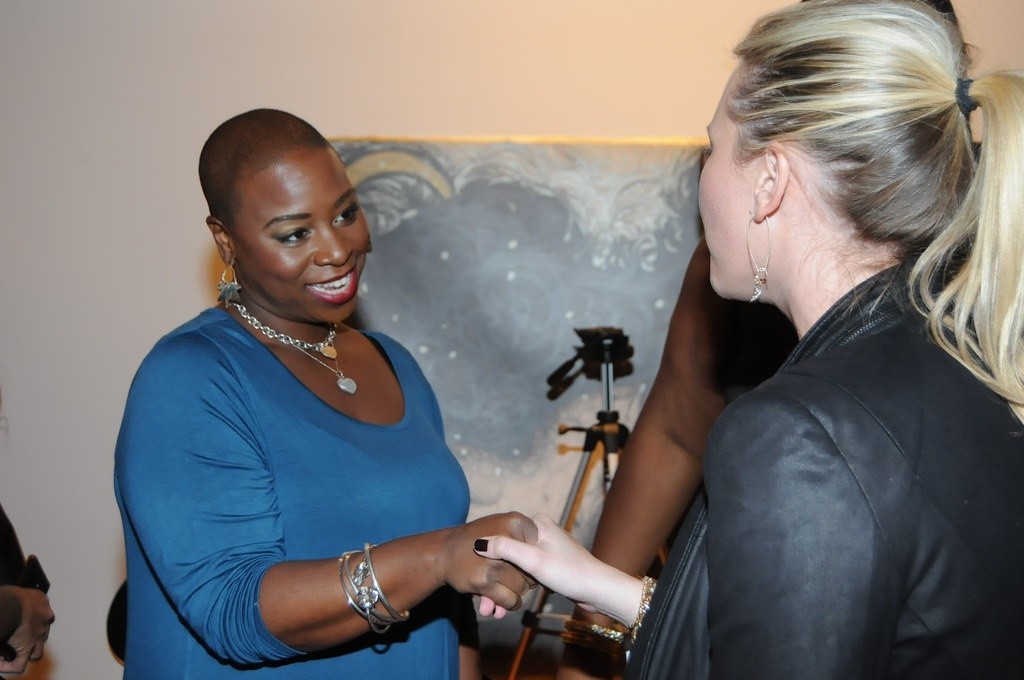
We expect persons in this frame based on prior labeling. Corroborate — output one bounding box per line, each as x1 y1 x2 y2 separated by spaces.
474 0 1024 680
112 108 537 680
0 506 55 680
556 0 959 680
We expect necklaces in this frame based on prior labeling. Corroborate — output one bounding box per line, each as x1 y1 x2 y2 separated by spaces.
231 301 357 396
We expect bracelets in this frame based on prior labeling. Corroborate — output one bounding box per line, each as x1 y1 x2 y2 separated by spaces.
335 542 409 635
561 574 659 658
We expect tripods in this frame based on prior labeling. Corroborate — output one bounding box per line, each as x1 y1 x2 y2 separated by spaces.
508 325 673 680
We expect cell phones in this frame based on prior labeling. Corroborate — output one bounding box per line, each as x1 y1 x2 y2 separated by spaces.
0 555 50 662
